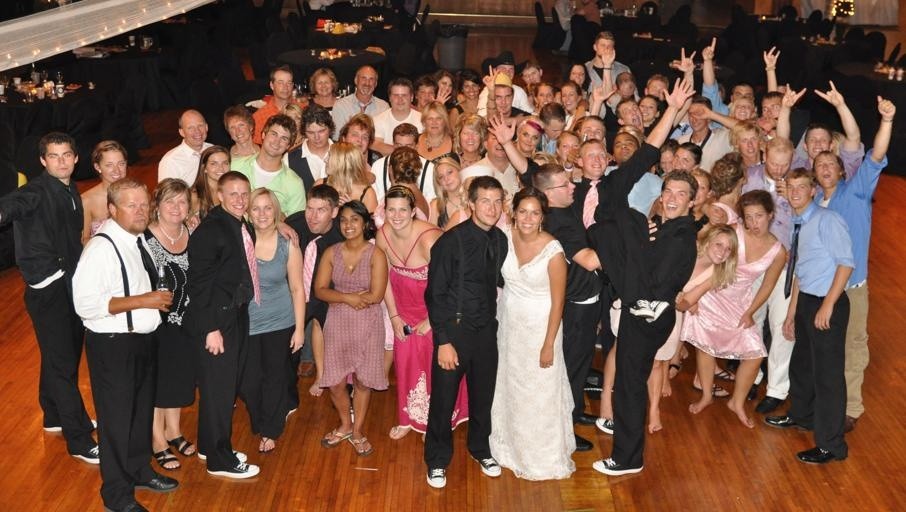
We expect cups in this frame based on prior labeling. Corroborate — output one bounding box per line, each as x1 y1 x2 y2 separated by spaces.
324 23 331 33
0 65 83 103
776 177 785 195
887 67 904 82
142 37 152 48
128 36 135 47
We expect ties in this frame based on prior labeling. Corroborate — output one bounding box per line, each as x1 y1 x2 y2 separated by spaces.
580 177 603 232
300 234 324 305
781 223 803 301
134 236 170 328
239 221 262 307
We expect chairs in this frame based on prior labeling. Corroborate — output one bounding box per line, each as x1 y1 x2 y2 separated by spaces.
533 2 906 179
187 0 439 107
1 20 187 196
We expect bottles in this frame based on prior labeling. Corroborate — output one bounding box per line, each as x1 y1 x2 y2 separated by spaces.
574 133 589 169
155 264 171 309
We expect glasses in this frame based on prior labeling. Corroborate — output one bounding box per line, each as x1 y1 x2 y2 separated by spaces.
542 180 569 191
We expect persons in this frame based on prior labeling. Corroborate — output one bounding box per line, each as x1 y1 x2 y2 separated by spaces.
1 131 102 467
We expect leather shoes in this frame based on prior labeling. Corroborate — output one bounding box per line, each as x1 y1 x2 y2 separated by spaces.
793 446 848 467
744 381 759 403
133 470 181 494
754 394 787 415
571 431 594 452
101 499 149 512
762 413 812 432
571 411 601 426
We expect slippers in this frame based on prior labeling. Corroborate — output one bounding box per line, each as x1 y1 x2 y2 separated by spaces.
321 426 355 449
348 432 375 456
711 366 737 381
388 423 413 441
257 435 276 455
689 383 730 398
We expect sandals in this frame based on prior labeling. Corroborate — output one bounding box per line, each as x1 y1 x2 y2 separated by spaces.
150 447 182 471
166 436 196 458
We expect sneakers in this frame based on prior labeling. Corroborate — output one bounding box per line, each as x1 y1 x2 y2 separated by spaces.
69 443 102 465
841 409 857 435
425 465 447 489
469 453 503 479
42 418 99 433
196 446 248 464
204 462 261 480
595 415 617 434
591 456 644 478
